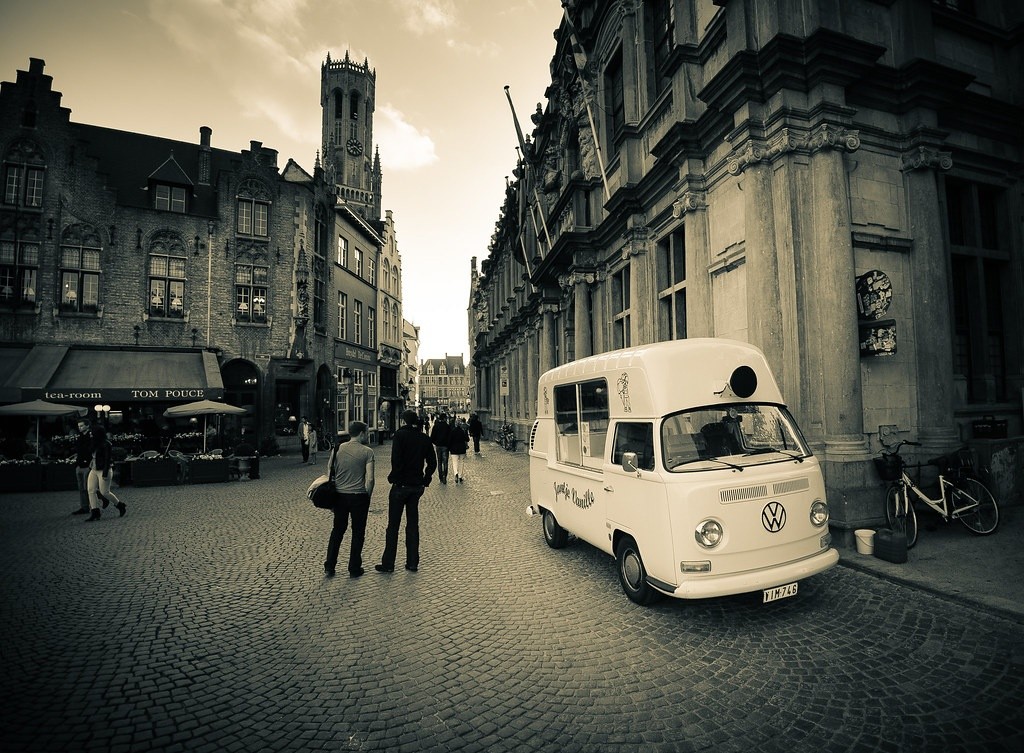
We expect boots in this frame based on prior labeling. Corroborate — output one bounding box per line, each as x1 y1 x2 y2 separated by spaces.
84 508 101 522
116 501 127 517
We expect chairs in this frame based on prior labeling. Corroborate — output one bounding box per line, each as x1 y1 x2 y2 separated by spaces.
700 423 740 457
619 425 654 470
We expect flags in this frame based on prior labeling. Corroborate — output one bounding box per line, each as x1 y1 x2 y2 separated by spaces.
564 5 592 83
505 90 538 266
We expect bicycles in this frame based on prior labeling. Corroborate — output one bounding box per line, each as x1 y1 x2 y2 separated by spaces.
872 433 999 548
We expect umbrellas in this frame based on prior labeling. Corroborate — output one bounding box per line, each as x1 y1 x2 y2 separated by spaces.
163 400 247 454
0 399 88 458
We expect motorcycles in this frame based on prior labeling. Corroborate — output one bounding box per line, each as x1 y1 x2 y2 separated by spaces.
500 422 516 451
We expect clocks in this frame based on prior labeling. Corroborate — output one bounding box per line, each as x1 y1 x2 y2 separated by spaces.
346 138 363 156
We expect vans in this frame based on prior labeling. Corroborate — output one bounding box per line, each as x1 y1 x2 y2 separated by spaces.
528 336 844 609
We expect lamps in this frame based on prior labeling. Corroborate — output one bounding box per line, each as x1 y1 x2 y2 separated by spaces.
409 378 413 384
343 368 351 389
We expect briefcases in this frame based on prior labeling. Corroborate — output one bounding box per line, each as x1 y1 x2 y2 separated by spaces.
972 415 1008 438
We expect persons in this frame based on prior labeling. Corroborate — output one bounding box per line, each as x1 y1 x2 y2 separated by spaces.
71 419 127 522
378 416 385 445
203 423 217 437
297 416 318 465
375 410 437 572
422 407 484 484
324 420 375 578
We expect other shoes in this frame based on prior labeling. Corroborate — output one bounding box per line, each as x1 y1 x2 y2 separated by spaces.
350 568 364 577
375 565 394 572
455 473 459 483
308 461 316 465
440 479 447 485
405 564 417 571
325 568 336 574
474 451 481 456
459 478 463 483
71 508 90 515
102 499 109 509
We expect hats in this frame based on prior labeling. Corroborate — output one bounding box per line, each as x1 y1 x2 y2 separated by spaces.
400 410 418 425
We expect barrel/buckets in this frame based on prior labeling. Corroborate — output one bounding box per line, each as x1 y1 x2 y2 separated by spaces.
854 529 876 555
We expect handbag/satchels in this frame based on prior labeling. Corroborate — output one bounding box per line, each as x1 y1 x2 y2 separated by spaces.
307 475 336 510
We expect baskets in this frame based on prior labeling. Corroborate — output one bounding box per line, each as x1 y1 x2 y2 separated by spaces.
873 454 902 481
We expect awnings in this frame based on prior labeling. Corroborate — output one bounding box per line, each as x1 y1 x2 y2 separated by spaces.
0 345 224 400
